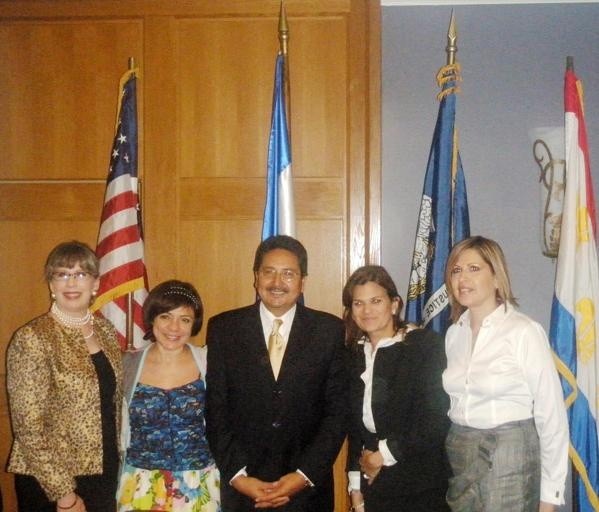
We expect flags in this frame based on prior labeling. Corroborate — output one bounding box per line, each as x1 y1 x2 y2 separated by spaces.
90 68 153 349
547 68 599 512
260 53 297 242
404 61 470 335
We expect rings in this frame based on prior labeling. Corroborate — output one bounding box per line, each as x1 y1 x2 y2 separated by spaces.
363 473 369 480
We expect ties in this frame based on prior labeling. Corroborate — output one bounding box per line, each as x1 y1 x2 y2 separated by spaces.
266 318 289 383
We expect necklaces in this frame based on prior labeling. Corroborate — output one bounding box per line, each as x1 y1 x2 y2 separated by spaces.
50 301 96 340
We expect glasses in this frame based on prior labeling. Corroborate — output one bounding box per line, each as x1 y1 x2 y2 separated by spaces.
50 270 97 284
254 267 307 283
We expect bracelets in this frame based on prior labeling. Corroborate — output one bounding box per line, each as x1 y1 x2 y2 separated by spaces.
304 478 312 488
59 494 78 510
347 503 365 512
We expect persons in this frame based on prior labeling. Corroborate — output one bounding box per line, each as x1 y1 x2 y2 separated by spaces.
7 240 122 512
118 279 222 512
343 266 454 511
442 235 568 512
207 234 345 512
